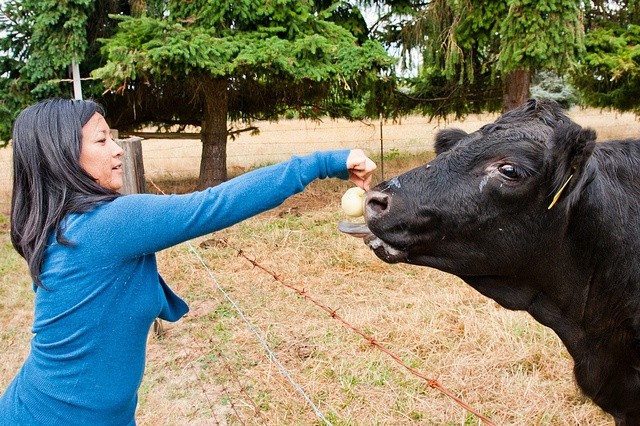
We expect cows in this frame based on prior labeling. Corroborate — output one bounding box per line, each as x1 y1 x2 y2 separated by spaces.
337 98 639 426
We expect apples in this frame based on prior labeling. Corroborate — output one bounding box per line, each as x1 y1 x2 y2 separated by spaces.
341 186 366 217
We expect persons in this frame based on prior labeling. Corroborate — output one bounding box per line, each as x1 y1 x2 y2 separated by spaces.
0 97 377 426
